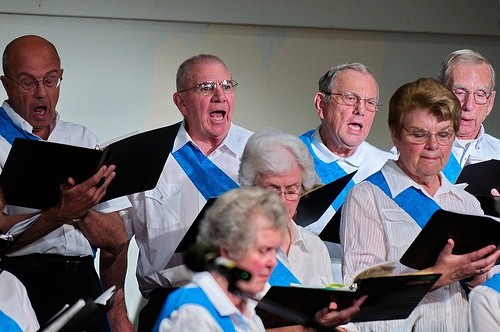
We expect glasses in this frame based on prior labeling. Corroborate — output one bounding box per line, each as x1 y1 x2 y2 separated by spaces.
259 186 302 201
323 92 383 112
402 124 455 146
7 74 62 91
451 88 493 104
179 80 239 96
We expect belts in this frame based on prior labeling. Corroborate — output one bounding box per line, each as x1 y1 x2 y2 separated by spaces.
12 260 93 274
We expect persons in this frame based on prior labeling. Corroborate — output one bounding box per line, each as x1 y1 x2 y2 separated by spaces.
292 49 500 332
0 35 360 332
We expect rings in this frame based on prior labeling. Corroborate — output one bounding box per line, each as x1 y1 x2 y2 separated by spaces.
480 268 484 274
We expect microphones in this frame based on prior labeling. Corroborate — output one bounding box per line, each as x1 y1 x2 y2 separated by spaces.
184 247 252 281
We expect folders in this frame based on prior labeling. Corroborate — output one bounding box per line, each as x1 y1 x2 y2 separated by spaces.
255 269 442 332
37 255 118 332
0 214 42 258
399 209 500 281
175 170 358 253
318 183 471 244
0 121 183 211
455 159 500 218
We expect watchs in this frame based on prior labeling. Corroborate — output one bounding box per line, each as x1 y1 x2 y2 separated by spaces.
70 210 89 226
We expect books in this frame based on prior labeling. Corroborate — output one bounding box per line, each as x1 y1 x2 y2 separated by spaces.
399 209 500 271
36 285 119 332
254 258 443 329
318 204 344 244
161 170 358 270
399 208 500 270
0 120 183 211
455 154 500 195
0 214 41 258
